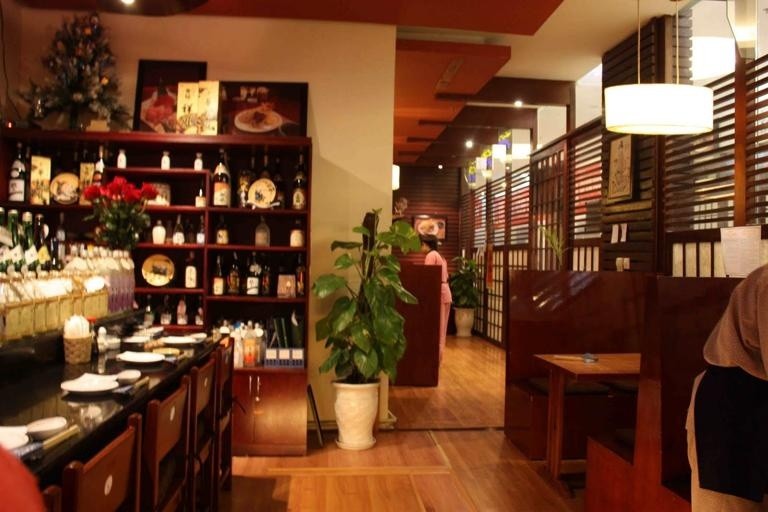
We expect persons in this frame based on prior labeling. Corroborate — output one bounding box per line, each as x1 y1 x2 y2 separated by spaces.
694 262 768 503
419 234 452 363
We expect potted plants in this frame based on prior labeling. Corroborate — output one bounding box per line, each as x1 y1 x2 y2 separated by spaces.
312 210 418 453
449 256 480 338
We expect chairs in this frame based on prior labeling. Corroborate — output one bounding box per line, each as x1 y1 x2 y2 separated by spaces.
217 336 235 497
43 485 62 512
191 350 218 512
144 374 193 512
61 413 144 512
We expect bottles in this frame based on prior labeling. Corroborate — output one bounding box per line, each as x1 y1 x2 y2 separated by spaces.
194 295 204 326
227 250 241 295
160 150 171 171
259 153 271 180
143 294 156 325
159 295 173 325
245 251 262 296
193 152 204 171
212 147 232 208
254 215 271 247
288 219 306 248
172 214 186 245
116 148 127 169
196 215 205 244
211 317 267 369
152 220 167 244
183 250 198 289
194 175 206 208
86 316 99 354
92 143 106 204
0 207 6 273
55 211 67 262
8 142 27 203
295 251 306 296
216 215 231 244
290 151 308 210
21 211 40 272
64 245 136 319
51 238 64 271
0 271 64 347
175 296 189 326
273 156 286 208
7 209 24 272
33 213 52 271
211 255 225 295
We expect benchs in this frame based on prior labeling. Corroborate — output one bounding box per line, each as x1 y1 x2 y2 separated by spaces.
504 267 654 461
648 274 746 511
583 275 648 511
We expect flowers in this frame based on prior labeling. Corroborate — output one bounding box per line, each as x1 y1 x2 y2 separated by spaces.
83 175 158 251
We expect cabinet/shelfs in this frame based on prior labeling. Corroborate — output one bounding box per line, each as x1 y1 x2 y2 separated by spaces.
1 124 311 457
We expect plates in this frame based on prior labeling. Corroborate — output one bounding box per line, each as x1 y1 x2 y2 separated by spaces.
162 336 195 344
1 426 29 450
234 108 283 133
50 173 80 204
248 177 277 204
119 352 164 364
141 255 175 287
61 379 119 394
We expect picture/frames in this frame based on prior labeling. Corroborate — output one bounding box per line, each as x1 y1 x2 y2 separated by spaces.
604 135 635 204
412 214 448 242
218 80 309 137
133 58 208 134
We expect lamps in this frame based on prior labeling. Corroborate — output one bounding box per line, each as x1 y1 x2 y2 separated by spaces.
604 1 715 137
